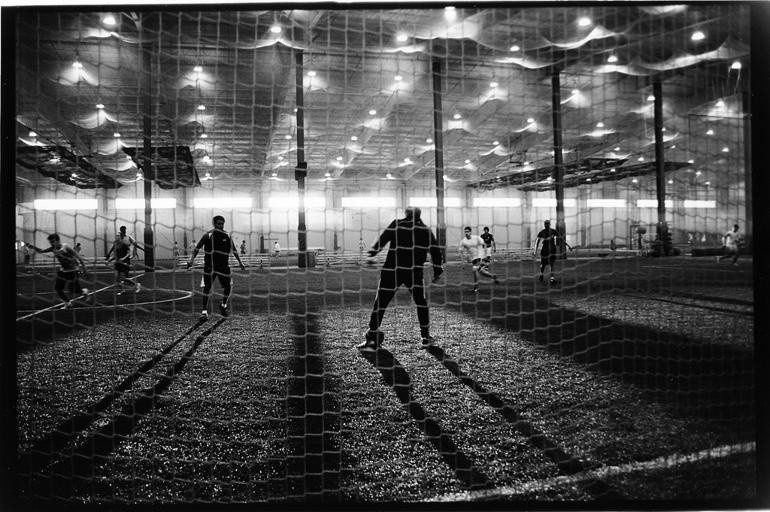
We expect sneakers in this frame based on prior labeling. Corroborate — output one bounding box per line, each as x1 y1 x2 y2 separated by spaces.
356 328 384 352
473 265 556 293
202 300 228 321
422 336 438 348
60 282 143 310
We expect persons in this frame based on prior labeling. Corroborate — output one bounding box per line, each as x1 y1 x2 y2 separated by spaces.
273 241 281 257
103 225 152 285
25 233 89 312
130 242 140 261
23 245 32 274
73 243 85 267
459 225 499 295
172 241 181 257
184 215 245 322
531 219 574 285
480 227 495 271
188 239 196 255
353 204 443 353
717 223 745 266
358 237 365 255
113 231 143 296
240 240 247 254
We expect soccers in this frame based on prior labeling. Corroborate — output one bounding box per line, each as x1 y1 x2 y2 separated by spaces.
366 328 384 345
